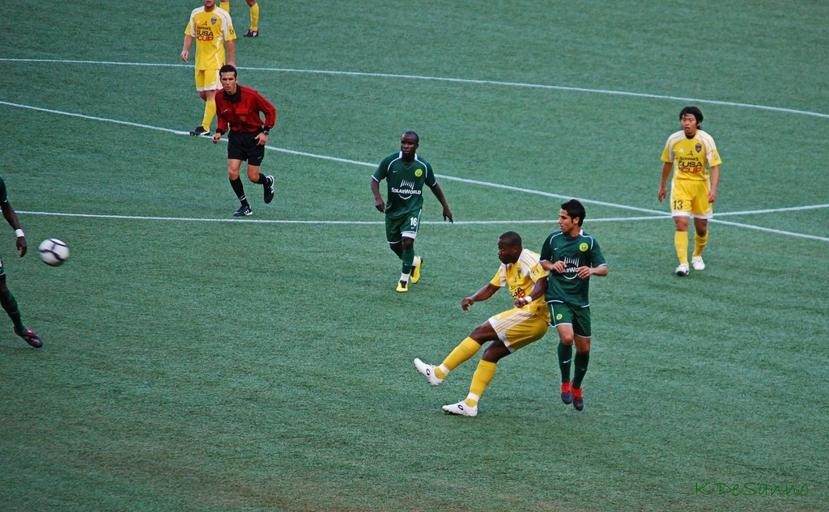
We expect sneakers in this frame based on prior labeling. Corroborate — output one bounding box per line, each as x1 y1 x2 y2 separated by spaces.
442 400 477 416
263 175 275 203
190 126 210 136
244 29 258 37
414 358 444 386
396 256 423 292
14 326 42 347
233 205 252 216
692 256 705 270
561 382 583 410
675 264 689 276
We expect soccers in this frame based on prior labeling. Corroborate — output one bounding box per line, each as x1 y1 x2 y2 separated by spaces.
37 238 70 267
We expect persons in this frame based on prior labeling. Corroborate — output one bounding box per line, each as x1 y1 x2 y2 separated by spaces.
212 65 276 216
0 178 44 349
413 231 550 417
181 1 237 136
370 130 453 292
219 0 259 37
658 106 722 276
539 199 609 411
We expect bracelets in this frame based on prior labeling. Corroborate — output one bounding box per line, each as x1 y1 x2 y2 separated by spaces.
14 228 23 237
524 295 532 303
